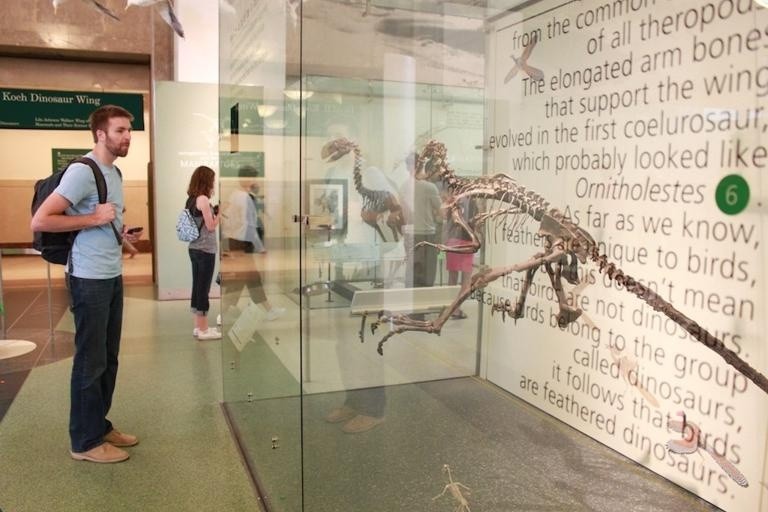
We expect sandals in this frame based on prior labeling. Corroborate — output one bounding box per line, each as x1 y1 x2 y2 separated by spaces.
450 310 467 318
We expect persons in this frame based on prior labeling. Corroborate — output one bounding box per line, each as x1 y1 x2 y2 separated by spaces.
216 165 286 326
322 120 400 435
245 182 264 253
399 152 443 321
445 199 479 320
184 166 228 340
30 105 138 464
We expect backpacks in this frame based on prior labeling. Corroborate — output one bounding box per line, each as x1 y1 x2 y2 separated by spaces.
176 197 205 241
32 157 122 265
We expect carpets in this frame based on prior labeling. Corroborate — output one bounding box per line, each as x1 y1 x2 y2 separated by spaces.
0 280 303 511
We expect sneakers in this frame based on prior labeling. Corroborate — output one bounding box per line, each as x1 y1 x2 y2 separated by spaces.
193 328 217 336
267 307 286 320
198 329 222 340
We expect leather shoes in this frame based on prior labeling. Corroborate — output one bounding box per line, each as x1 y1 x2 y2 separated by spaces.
105 428 138 446
342 415 385 433
326 407 356 422
71 442 129 463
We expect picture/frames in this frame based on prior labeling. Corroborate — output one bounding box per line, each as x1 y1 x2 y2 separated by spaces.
304 179 347 231
